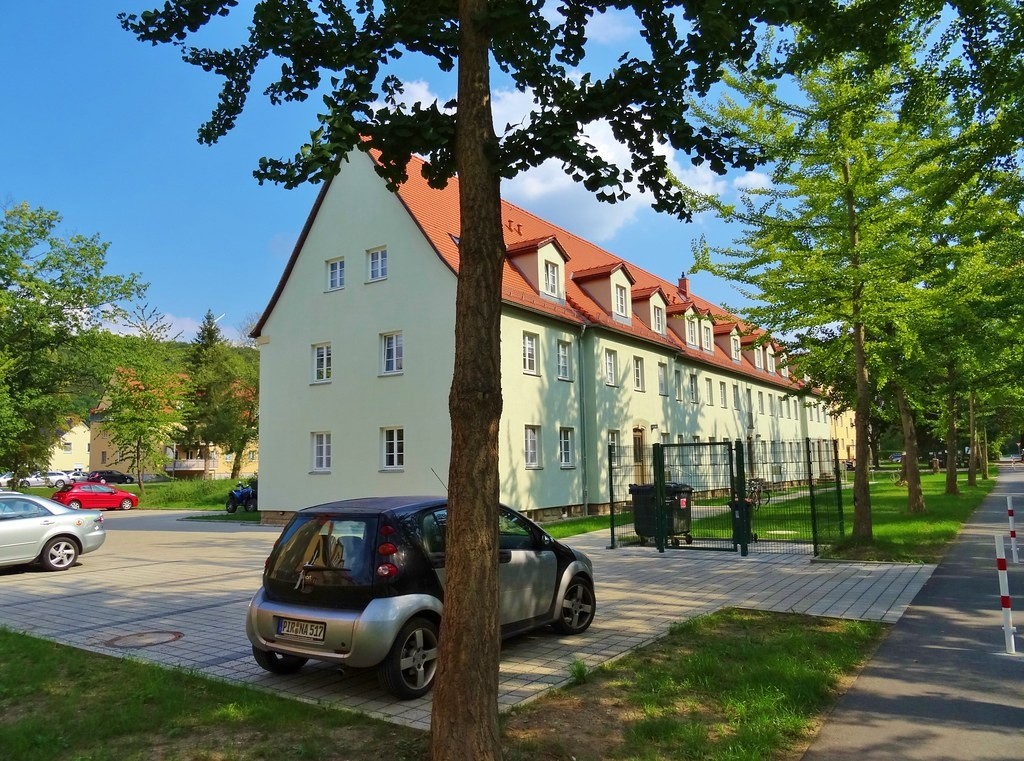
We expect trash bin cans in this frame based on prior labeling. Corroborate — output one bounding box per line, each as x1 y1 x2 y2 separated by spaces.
628 483 694 548
727 499 759 545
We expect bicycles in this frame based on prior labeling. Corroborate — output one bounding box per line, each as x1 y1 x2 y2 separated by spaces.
744 478 770 511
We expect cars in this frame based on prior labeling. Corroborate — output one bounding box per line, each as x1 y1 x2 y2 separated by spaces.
50 482 139 512
18 470 70 489
87 469 134 486
62 471 90 484
1 491 107 571
243 494 597 700
0 472 23 488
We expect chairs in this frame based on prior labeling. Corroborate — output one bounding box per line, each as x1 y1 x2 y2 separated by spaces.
319 534 336 565
337 536 361 559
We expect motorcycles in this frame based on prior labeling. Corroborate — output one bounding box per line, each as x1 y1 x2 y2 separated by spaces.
226 483 258 514
